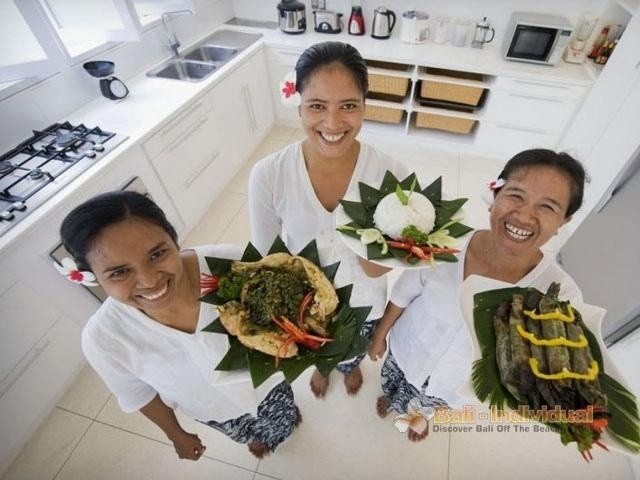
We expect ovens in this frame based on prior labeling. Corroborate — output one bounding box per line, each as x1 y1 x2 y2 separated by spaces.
48 175 174 307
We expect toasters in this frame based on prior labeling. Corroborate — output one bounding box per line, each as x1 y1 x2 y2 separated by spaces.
312 10 343 34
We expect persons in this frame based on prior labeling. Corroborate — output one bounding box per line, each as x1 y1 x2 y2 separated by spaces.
248 42 410 399
61 191 302 460
369 149 585 441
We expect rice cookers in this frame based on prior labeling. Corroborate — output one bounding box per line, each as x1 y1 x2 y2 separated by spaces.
276 0 307 34
401 11 432 44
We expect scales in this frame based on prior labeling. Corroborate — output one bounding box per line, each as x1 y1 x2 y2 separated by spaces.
84 61 129 101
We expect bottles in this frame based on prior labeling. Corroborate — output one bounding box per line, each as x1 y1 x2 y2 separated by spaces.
587 21 627 65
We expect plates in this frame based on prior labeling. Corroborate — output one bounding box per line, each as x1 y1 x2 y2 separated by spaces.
457 274 638 415
335 180 473 270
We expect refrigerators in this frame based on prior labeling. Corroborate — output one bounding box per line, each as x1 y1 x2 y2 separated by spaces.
556 144 640 350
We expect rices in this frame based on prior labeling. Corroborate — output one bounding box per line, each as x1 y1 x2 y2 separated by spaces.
373 189 436 242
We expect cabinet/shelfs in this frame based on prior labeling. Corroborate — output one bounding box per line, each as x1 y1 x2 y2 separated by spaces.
0 50 275 479
266 46 498 145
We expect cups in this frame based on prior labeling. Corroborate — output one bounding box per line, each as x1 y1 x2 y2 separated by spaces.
436 14 472 48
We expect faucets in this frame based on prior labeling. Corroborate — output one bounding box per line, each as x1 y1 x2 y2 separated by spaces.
161 8 197 42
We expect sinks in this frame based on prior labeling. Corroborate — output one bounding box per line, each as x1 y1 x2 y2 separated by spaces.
156 61 217 82
185 45 238 61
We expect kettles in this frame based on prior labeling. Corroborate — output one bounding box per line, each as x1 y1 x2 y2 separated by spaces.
371 6 396 39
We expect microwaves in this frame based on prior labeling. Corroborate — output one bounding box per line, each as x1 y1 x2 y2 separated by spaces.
501 12 573 67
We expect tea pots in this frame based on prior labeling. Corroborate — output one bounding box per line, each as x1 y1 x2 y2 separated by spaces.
472 16 494 48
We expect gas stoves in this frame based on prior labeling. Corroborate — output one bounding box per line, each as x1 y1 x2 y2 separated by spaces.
0 121 116 232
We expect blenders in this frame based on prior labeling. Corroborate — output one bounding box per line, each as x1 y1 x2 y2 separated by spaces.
562 14 599 65
350 0 365 36
